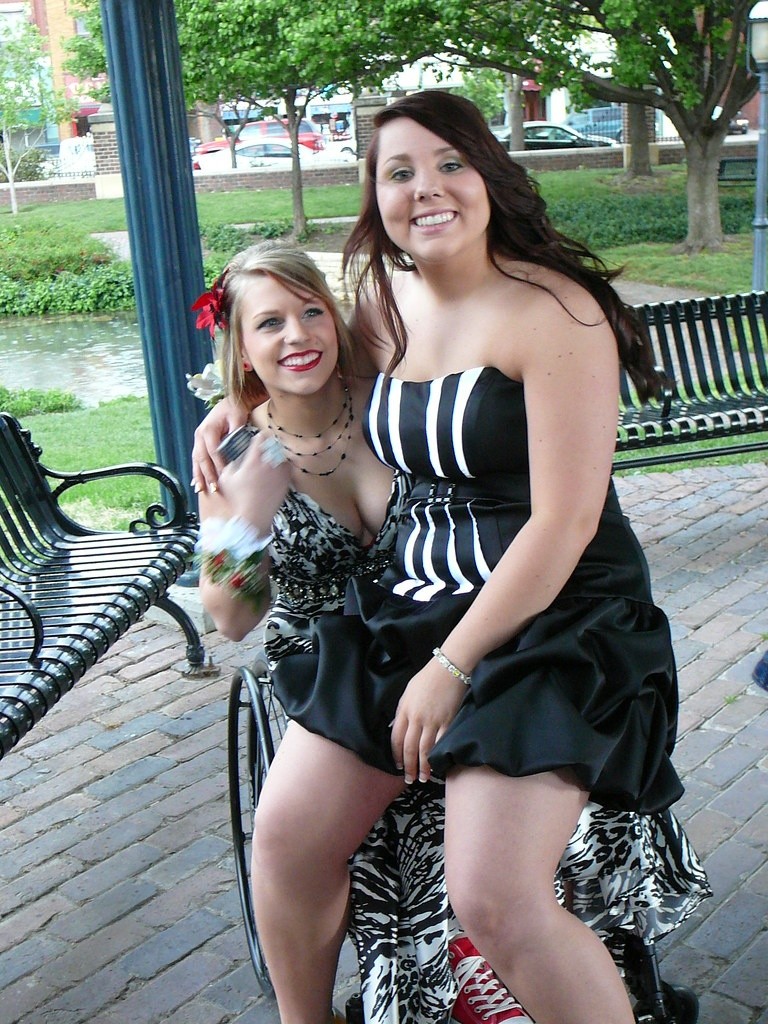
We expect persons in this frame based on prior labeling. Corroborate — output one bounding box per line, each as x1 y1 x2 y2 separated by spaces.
190 90 688 1023
192 238 717 1023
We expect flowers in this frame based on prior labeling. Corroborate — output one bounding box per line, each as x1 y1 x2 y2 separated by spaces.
191 280 227 339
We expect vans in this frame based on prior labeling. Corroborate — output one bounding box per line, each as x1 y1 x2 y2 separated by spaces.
34 144 60 159
555 106 664 143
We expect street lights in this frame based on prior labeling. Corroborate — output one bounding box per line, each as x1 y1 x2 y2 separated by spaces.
746 1 768 292
36 64 49 145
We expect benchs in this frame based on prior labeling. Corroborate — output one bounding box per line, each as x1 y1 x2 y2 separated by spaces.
611 290 768 475
0 411 220 764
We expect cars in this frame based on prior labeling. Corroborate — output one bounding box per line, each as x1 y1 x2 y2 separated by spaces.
334 139 357 162
491 121 618 152
713 109 749 134
191 119 326 156
191 138 324 171
327 120 352 142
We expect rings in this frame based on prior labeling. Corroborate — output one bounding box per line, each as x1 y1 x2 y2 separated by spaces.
258 437 285 468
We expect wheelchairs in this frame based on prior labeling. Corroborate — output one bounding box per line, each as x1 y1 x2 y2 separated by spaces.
228 651 700 1024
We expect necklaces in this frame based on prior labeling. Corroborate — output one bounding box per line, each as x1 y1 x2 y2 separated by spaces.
267 377 354 477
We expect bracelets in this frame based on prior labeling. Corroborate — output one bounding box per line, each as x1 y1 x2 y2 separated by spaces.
431 646 472 686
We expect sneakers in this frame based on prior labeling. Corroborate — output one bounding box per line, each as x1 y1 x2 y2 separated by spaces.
448 932 537 1024
